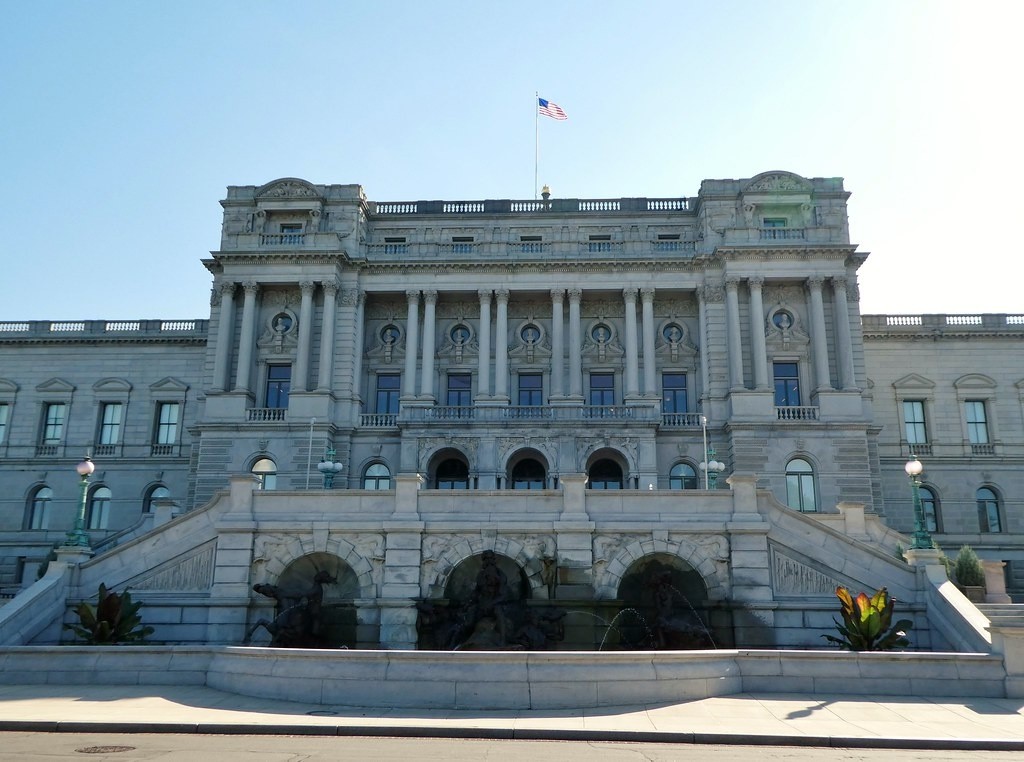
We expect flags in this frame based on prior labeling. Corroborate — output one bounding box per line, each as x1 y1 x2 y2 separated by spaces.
539 97 568 120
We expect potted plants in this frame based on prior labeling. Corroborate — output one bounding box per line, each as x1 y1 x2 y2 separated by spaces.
955 544 986 603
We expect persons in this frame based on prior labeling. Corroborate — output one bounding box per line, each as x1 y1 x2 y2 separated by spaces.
448 549 515 650
276 569 338 639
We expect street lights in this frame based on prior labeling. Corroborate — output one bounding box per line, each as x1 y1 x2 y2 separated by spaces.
905 453 936 551
59 454 97 548
317 459 345 489
305 415 318 487
699 415 711 490
699 459 725 492
